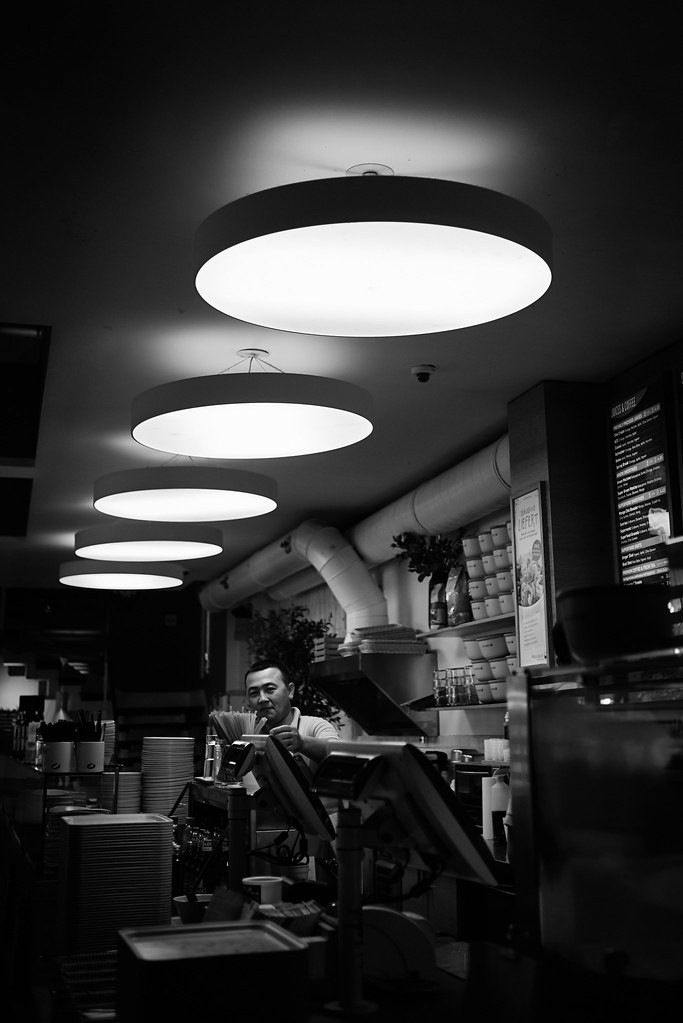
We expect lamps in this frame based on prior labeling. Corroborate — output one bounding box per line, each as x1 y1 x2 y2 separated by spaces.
59 557 187 592
91 465 279 527
70 519 226 564
175 170 562 341
128 366 378 466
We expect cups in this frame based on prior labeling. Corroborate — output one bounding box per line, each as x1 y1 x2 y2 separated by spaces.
75 741 106 772
44 741 75 773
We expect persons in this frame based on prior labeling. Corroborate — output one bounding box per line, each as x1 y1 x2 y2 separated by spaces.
243 658 341 857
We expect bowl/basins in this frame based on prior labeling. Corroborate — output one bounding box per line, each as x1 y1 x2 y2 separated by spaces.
433 518 519 707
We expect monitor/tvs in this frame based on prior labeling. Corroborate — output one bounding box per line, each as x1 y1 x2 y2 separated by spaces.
397 744 503 886
261 736 336 841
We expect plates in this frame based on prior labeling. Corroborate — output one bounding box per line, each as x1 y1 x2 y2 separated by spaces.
101 737 195 827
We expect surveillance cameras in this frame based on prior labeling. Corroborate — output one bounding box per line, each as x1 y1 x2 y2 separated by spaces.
182 569 191 576
411 365 436 383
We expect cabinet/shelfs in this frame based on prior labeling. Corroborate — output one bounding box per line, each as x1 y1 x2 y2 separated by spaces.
406 607 517 717
